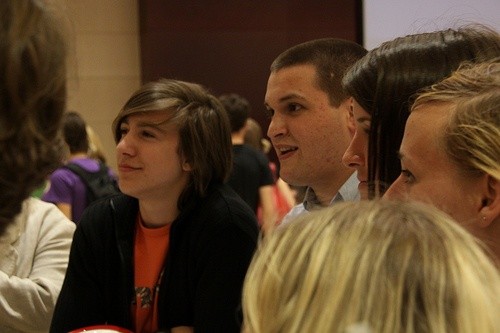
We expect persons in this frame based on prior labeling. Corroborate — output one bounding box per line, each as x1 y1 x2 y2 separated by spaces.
48 81 262 333
0 0 500 333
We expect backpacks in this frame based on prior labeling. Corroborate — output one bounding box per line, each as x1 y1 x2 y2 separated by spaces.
62 158 122 211
